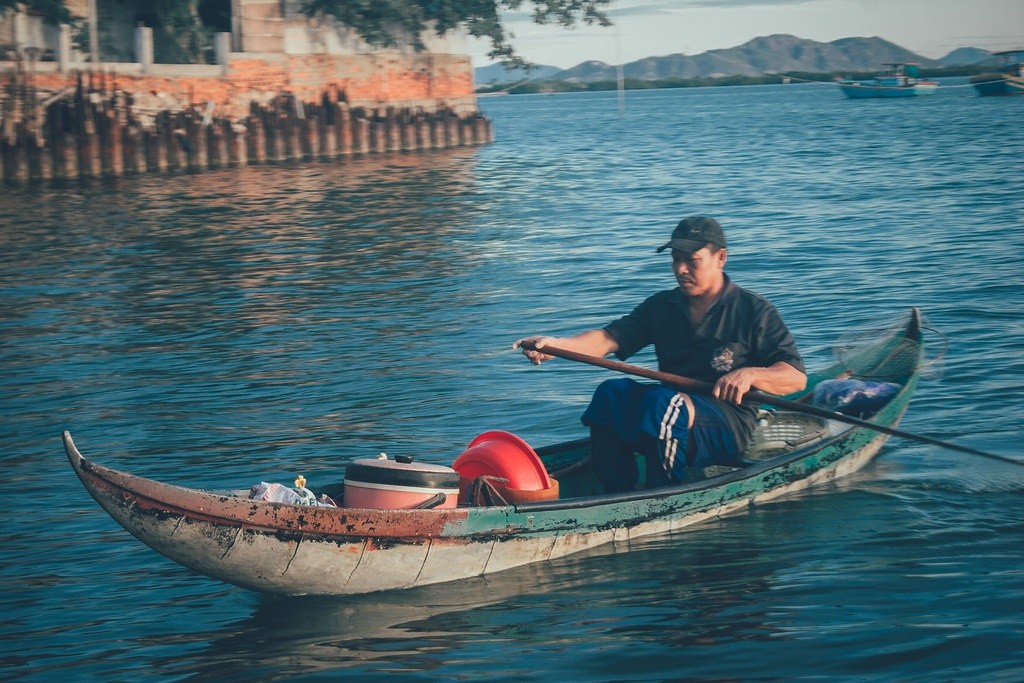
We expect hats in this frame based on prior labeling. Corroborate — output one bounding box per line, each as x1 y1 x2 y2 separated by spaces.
656 215 727 254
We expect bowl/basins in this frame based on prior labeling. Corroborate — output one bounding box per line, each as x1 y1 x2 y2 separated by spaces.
451 430 551 490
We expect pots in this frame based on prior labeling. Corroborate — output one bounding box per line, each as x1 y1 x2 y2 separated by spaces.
344 455 460 509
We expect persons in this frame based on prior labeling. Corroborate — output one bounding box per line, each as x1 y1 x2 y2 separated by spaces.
513 215 809 493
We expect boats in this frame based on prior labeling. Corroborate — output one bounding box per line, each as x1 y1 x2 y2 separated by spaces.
63 306 926 598
837 62 940 97
970 49 1024 96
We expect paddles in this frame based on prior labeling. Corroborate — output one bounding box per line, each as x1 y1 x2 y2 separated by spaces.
522 340 1023 468
